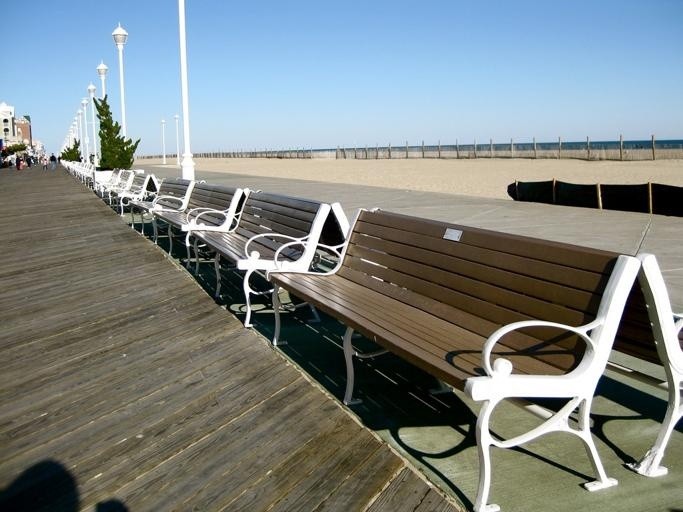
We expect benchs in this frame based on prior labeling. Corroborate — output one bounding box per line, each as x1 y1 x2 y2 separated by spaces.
55 157 240 268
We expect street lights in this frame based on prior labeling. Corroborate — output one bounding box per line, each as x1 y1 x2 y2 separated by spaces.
159 120 167 165
97 59 108 104
112 21 128 151
87 82 97 167
61 98 89 166
174 115 182 169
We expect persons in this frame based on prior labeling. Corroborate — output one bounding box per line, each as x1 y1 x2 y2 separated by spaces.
7 153 60 171
